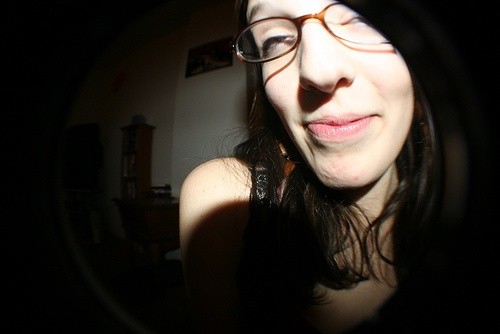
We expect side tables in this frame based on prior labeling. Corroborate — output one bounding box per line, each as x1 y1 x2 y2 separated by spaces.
114 196 181 259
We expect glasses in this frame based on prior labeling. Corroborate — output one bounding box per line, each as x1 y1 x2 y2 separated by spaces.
232 3 392 64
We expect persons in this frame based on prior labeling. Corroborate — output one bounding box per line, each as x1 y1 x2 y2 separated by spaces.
180 0 485 333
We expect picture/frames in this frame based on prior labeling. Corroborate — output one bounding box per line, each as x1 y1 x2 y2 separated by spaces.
185 34 233 78
